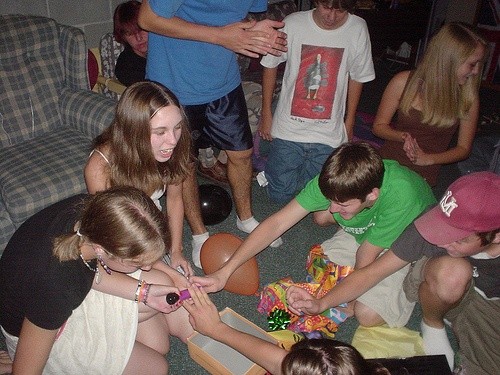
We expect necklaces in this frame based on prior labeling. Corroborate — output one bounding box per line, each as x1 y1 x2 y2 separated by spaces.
79 253 102 286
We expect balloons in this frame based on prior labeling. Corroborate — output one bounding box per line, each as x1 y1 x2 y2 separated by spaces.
200 233 260 299
196 184 233 225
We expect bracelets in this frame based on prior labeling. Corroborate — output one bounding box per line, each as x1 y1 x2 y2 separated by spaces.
134 280 152 305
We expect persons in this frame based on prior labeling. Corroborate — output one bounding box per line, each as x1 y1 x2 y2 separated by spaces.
186 139 444 331
285 170 499 374
81 78 199 356
180 279 397 374
0 184 178 375
257 1 377 207
368 20 490 200
137 1 288 271
112 0 262 185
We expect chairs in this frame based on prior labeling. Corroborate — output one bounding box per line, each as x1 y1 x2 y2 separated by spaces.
96 33 127 101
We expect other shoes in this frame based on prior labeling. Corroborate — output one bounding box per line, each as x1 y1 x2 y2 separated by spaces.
197 160 231 186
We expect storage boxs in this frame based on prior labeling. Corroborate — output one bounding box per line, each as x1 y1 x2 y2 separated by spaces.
187 307 280 375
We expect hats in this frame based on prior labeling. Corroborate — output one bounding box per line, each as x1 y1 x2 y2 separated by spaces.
414 171 500 245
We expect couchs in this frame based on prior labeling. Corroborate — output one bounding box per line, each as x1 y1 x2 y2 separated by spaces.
0 14 119 259
